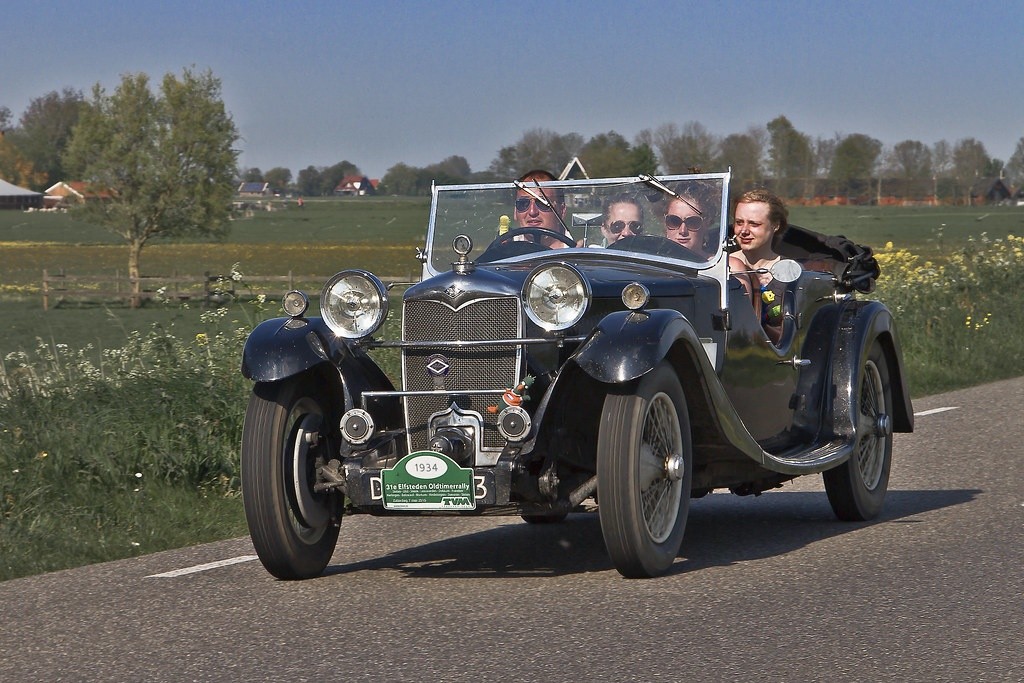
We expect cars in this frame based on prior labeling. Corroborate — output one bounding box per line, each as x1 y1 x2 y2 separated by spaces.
239 167 916 580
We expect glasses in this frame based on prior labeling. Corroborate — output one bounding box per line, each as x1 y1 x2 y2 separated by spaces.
664 214 707 231
605 220 643 234
515 196 558 212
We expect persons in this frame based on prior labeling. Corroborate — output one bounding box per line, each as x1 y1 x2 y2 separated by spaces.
584 193 647 251
487 169 583 250
656 168 754 306
729 189 805 343
